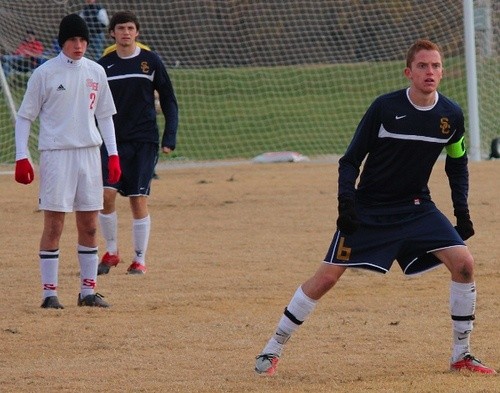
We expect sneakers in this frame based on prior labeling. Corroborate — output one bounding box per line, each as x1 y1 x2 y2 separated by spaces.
253 338 286 376
77 292 111 309
126 261 148 274
97 248 120 275
448 353 497 375
39 295 65 309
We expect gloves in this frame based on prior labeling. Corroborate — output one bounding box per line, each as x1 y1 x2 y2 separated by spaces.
15 159 34 184
452 210 476 240
336 198 358 237
106 154 121 184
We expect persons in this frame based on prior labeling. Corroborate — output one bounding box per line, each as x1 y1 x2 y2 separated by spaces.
79 0 109 61
255 41 495 376
15 14 121 309
95 9 179 274
10 30 44 72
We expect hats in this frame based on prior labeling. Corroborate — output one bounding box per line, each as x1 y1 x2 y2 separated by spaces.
58 13 89 49
